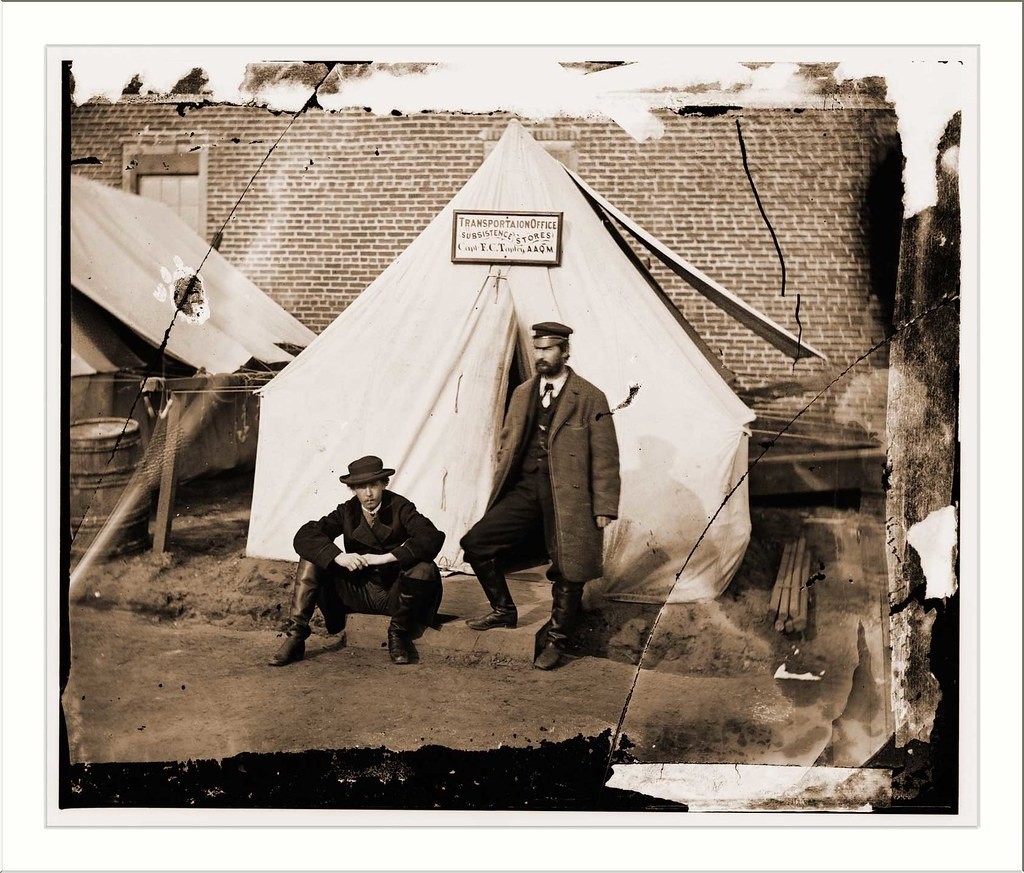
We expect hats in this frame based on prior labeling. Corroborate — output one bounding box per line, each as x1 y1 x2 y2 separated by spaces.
531 322 574 348
339 455 396 485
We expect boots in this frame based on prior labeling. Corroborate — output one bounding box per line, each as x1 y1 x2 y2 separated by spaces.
387 573 433 664
465 558 518 630
268 557 325 665
535 584 586 670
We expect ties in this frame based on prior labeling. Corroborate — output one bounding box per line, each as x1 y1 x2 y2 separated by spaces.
542 383 554 409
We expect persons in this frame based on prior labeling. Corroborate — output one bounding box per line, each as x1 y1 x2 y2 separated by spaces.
462 321 622 670
265 456 445 663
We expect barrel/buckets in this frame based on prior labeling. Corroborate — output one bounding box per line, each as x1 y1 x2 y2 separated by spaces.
70 416 152 567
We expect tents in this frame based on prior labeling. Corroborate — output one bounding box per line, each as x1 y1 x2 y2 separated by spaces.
243 120 827 603
63 172 320 484
